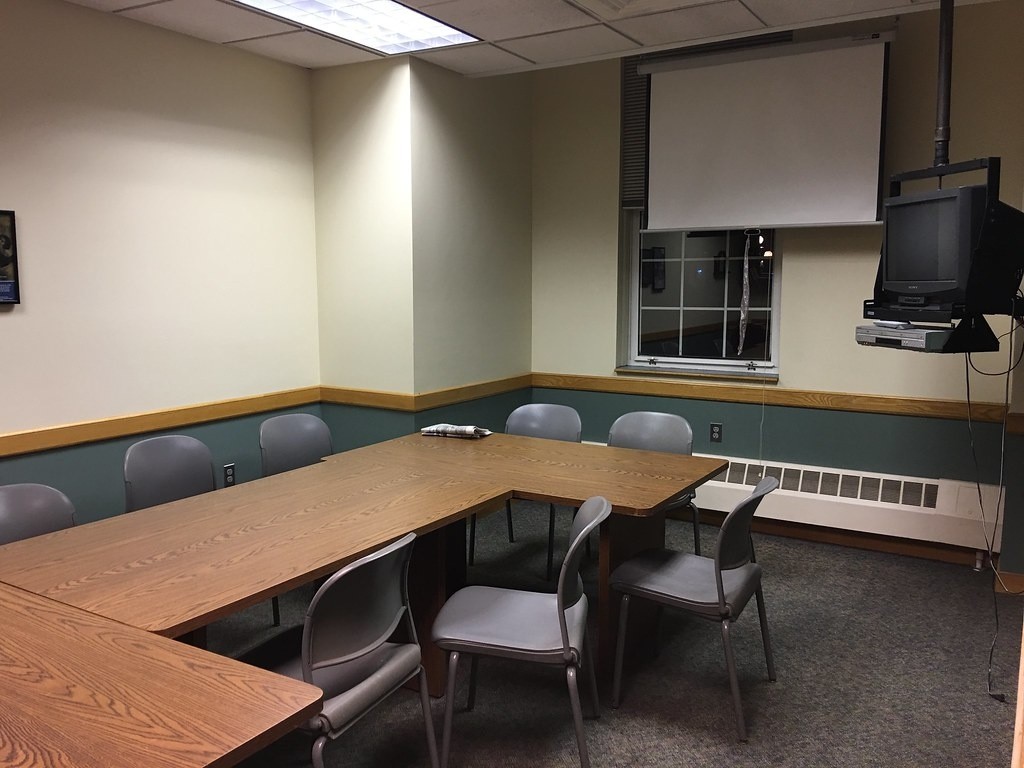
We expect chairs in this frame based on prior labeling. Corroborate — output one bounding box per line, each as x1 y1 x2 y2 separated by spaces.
259 413 332 600
0 483 75 544
228 533 440 768
429 496 612 768
607 477 782 743
469 403 583 582
124 434 281 624
572 411 701 562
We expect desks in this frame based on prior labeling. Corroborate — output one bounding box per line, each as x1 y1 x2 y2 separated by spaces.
0 459 513 697
0 582 323 768
321 431 729 676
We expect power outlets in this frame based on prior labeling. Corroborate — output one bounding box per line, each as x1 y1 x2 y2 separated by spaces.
710 423 723 443
224 462 236 488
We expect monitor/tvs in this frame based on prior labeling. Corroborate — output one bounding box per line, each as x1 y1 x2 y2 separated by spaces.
881 185 1024 312
